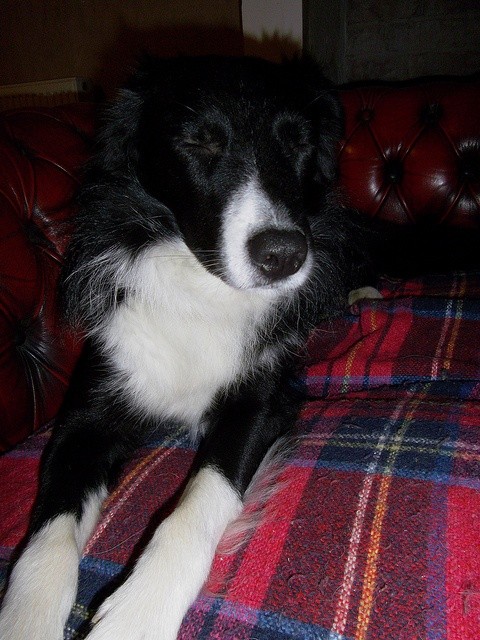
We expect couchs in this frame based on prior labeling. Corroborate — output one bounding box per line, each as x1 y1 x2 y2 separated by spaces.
0 75 480 635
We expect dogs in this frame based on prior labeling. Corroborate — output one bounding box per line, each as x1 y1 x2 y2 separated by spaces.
0 30 353 640
